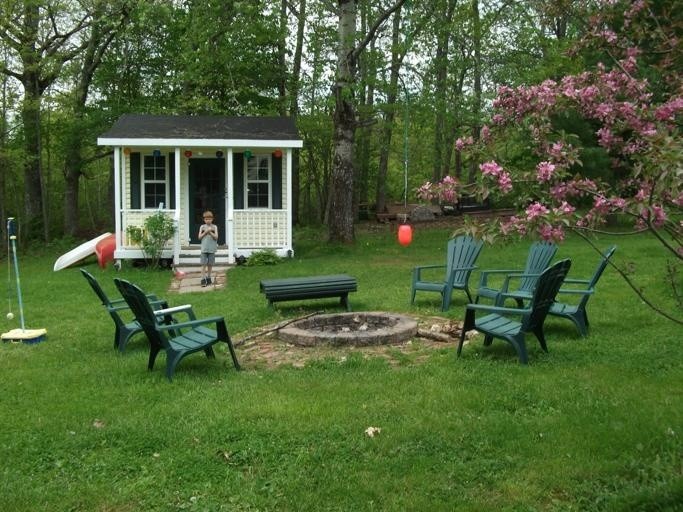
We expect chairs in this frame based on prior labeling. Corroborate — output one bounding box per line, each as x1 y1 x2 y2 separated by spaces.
411 235 484 314
456 257 572 365
113 278 239 383
474 240 559 307
522 244 618 338
79 267 182 352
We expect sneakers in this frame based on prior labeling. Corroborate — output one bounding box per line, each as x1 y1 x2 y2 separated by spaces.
201 278 211 287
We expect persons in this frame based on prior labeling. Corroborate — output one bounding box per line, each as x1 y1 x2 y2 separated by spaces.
197 210 218 287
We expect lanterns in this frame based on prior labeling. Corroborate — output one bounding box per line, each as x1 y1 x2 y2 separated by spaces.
274 149 281 158
184 150 191 159
153 149 160 158
121 147 130 156
243 150 251 160
397 223 412 247
215 150 223 159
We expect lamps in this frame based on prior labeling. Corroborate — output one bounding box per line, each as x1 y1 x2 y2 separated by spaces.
216 148 223 157
244 147 251 158
153 149 160 157
185 148 192 157
124 147 131 155
274 148 282 158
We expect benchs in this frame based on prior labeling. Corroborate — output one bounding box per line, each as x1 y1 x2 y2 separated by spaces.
260 274 357 312
